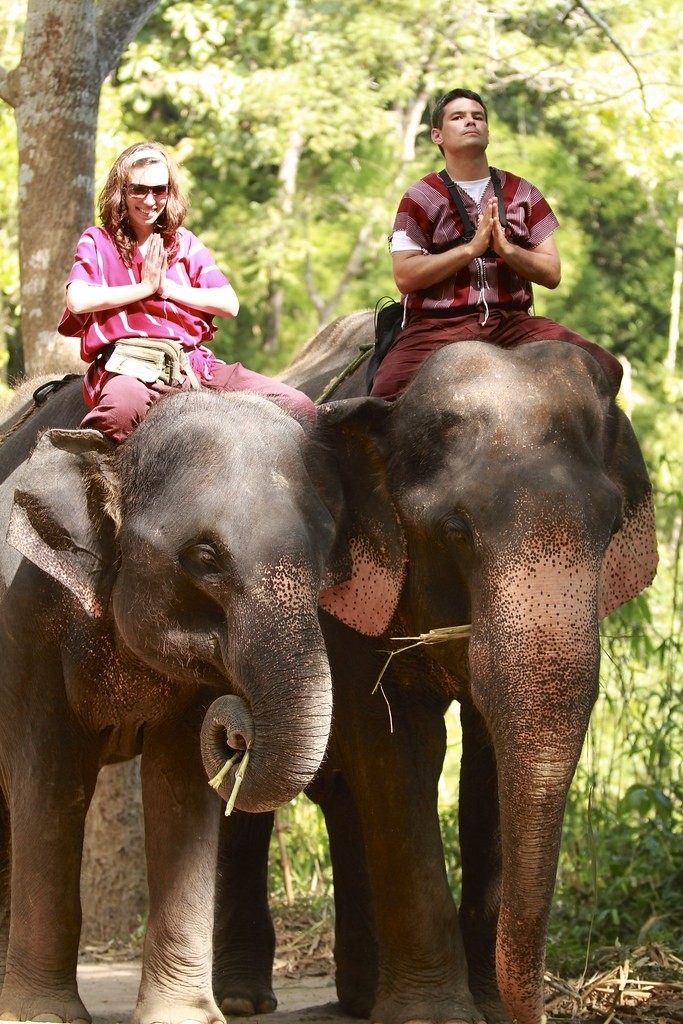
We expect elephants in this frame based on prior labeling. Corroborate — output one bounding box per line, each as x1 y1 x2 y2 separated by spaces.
0 370 407 1023
211 306 659 1024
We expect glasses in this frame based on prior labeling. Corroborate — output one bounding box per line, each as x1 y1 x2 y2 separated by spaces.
126 183 171 199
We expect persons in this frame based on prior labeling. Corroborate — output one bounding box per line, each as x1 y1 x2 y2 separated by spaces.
56 142 318 448
366 88 623 402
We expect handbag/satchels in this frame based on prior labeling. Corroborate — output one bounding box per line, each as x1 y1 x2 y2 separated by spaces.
105 337 202 391
